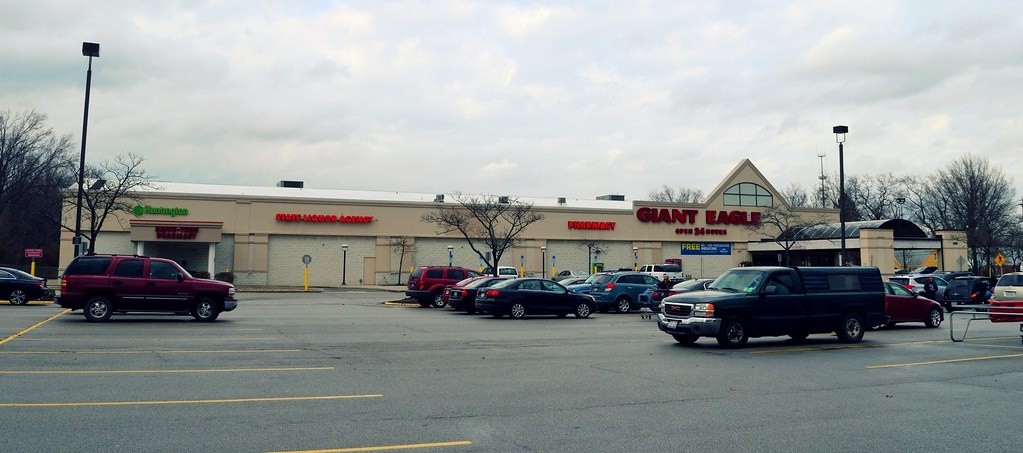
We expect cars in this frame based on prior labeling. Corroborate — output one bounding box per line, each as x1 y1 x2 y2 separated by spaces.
648 278 718 311
448 269 669 319
881 265 1023 329
0 266 49 305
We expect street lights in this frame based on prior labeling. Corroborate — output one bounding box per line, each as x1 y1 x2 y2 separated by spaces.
71 42 102 256
832 125 849 267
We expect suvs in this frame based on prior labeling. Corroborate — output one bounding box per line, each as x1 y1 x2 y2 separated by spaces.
53 252 237 322
658 266 888 350
404 264 485 308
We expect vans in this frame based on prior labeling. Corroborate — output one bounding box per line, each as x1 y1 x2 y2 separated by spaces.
638 264 684 285
481 267 520 279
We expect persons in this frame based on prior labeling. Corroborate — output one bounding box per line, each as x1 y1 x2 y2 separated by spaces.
988 275 998 288
925 277 938 301
654 275 671 289
